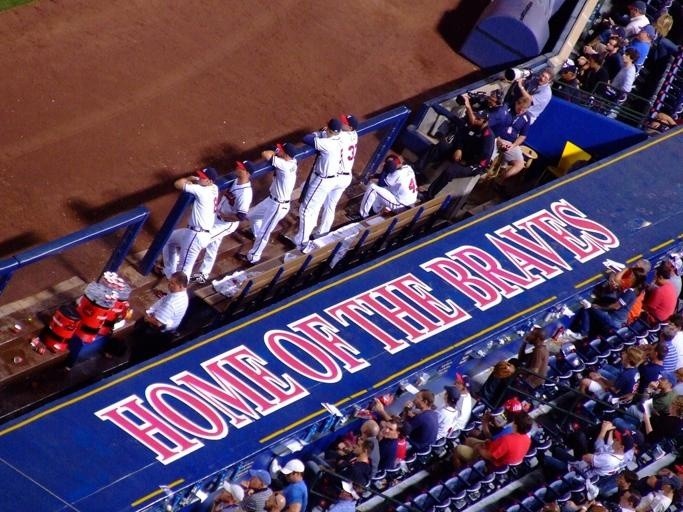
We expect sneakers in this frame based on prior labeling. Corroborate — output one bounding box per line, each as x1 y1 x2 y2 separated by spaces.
189 273 206 284
152 264 162 276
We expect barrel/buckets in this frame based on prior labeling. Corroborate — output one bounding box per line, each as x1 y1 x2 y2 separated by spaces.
38 270 131 354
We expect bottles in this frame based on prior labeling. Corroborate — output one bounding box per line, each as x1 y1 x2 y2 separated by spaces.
477 302 567 362
85 270 132 307
651 248 683 275
162 459 243 512
305 371 432 440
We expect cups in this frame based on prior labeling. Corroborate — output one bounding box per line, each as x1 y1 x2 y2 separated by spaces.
12 314 62 364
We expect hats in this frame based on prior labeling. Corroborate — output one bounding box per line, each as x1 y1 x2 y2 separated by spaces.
455 372 472 388
195 167 217 181
637 24 655 39
327 116 341 132
276 142 296 156
502 399 522 414
627 1 646 10
339 113 357 129
443 386 459 403
222 459 304 504
235 160 255 175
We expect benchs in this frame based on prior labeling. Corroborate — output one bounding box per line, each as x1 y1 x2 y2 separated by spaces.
192 189 454 322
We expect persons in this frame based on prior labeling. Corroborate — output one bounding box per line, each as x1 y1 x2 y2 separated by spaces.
192 159 254 285
345 153 420 223
129 270 188 365
311 113 357 239
178 382 683 511
238 141 298 266
490 249 683 379
152 167 220 284
293 117 341 247
422 3 681 199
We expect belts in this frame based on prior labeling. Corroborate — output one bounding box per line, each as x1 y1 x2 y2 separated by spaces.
337 172 349 174
187 225 209 232
269 195 289 203
217 215 227 221
314 171 334 178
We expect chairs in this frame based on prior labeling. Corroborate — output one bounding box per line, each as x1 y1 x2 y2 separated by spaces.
533 140 592 188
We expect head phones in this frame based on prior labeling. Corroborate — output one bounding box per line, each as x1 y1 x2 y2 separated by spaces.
483 113 489 123
495 88 501 105
